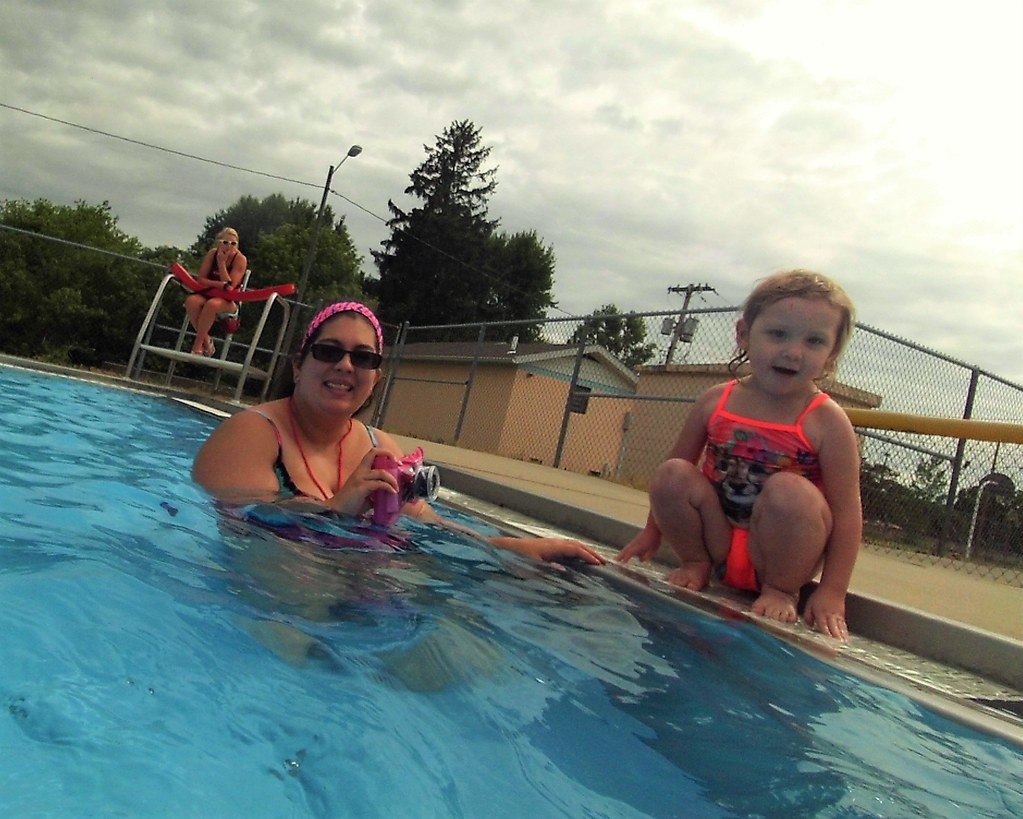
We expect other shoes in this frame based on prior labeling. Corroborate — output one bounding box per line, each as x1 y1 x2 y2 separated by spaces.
191 338 215 357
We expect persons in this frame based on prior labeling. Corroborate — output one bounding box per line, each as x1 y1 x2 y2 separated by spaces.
192 301 610 566
187 227 247 357
627 269 863 639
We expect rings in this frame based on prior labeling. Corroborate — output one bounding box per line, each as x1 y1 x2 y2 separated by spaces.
218 253 219 255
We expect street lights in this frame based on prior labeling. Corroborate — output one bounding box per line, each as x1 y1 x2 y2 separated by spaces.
269 144 363 403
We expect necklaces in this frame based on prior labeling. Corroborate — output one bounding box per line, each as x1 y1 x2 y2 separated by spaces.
288 396 352 500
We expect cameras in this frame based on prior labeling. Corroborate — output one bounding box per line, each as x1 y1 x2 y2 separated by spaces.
372 447 440 527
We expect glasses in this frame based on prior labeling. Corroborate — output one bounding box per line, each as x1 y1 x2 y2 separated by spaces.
310 340 382 370
220 240 238 246
